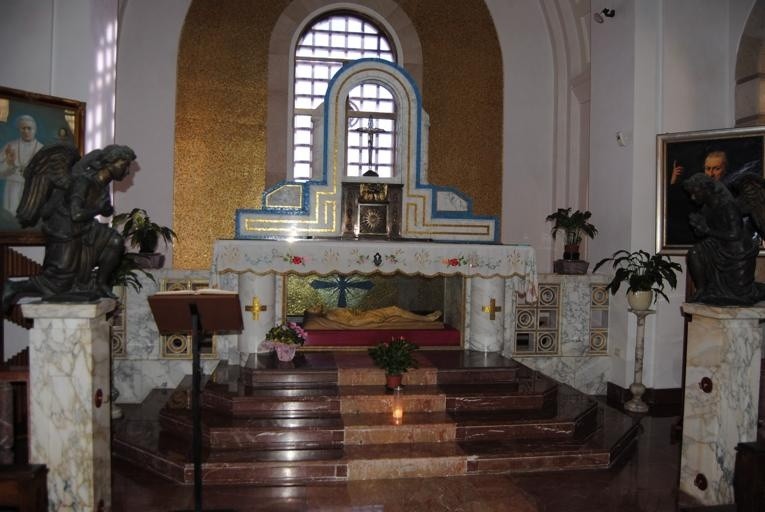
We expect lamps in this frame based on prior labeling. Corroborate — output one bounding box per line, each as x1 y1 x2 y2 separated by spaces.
593 8 615 23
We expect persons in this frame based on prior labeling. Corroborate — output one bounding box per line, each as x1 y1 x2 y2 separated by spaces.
681 161 765 309
1 143 135 317
301 300 445 329
0 113 48 221
667 147 726 243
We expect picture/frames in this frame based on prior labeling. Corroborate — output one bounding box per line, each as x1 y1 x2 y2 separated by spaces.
1 86 87 246
654 126 764 256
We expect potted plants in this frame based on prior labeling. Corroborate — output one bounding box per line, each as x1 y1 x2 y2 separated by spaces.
545 207 598 261
591 249 682 310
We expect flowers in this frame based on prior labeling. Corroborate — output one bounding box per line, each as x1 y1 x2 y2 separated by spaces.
265 320 311 347
368 336 419 374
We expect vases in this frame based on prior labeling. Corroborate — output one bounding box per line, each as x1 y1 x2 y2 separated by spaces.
274 344 296 362
384 374 402 390
